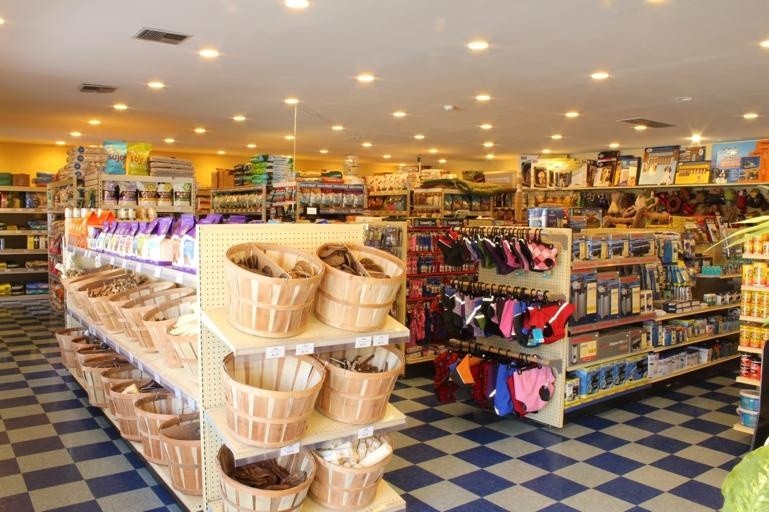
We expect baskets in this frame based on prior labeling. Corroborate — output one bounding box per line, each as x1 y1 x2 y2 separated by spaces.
142 295 198 368
53 327 102 368
75 345 116 387
308 344 406 425
107 281 177 342
215 444 318 512
314 242 408 334
220 351 327 449
223 241 326 339
108 380 167 442
79 355 134 408
132 393 179 466
307 432 394 511
157 412 203 496
163 314 199 378
86 275 152 334
120 287 195 352
57 262 126 325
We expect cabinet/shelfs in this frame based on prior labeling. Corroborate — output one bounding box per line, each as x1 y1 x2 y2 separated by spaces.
515 182 767 218
429 217 745 428
208 180 370 222
42 174 197 313
733 209 769 436
64 202 410 512
362 185 514 368
0 184 48 301
